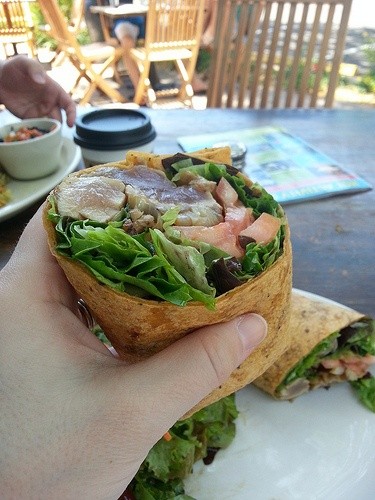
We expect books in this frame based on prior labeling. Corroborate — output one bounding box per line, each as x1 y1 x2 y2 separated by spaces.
177 125 372 206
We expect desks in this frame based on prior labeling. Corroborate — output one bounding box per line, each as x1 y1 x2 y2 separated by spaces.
0 107 375 316
89 5 146 85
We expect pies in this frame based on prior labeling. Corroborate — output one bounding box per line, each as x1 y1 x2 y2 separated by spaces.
253 288 375 411
41 146 290 422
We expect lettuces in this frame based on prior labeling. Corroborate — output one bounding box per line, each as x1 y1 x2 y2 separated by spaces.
124 392 239 500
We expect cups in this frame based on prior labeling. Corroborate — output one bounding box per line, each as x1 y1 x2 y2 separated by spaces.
213 140 247 173
72 107 159 168
0 118 63 180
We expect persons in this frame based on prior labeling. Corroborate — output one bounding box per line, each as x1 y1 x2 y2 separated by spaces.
84 0 209 106
0 200 267 500
0 55 76 127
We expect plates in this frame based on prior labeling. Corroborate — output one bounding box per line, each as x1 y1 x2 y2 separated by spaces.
0 106 83 222
108 345 375 499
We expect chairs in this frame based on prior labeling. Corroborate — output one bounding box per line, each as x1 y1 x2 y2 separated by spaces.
0 0 353 110
